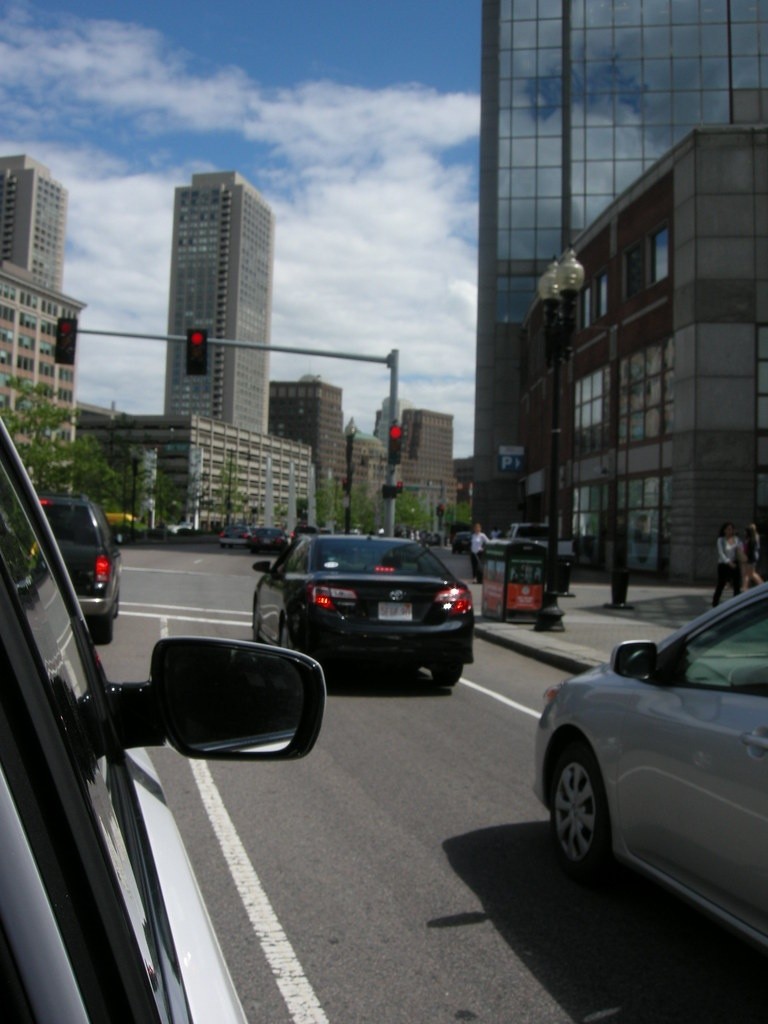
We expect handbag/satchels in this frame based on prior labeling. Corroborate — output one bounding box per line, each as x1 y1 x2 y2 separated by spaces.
735 537 747 562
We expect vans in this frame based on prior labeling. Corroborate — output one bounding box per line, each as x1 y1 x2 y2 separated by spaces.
291 524 321 539
506 523 549 541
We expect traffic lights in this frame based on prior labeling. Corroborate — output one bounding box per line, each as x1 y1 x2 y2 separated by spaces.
186 328 209 375
54 318 78 365
388 425 403 464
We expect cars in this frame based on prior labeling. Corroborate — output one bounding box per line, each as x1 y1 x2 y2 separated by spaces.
0 413 326 1022
536 251 588 633
528 579 767 952
451 531 472 554
247 527 292 555
250 533 476 690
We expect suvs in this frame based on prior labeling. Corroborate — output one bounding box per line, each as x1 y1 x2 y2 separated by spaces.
220 525 254 548
36 491 123 645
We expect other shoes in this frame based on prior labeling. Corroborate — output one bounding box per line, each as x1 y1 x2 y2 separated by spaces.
473 578 482 584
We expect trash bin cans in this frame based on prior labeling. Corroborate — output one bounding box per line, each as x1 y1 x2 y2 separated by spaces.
481 537 548 625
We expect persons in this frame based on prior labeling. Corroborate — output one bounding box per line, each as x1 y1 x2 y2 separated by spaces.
712 521 747 608
471 523 490 584
572 532 582 570
740 523 764 592
489 526 503 540
419 529 427 547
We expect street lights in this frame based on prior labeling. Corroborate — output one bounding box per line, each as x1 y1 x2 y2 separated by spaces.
344 416 357 534
